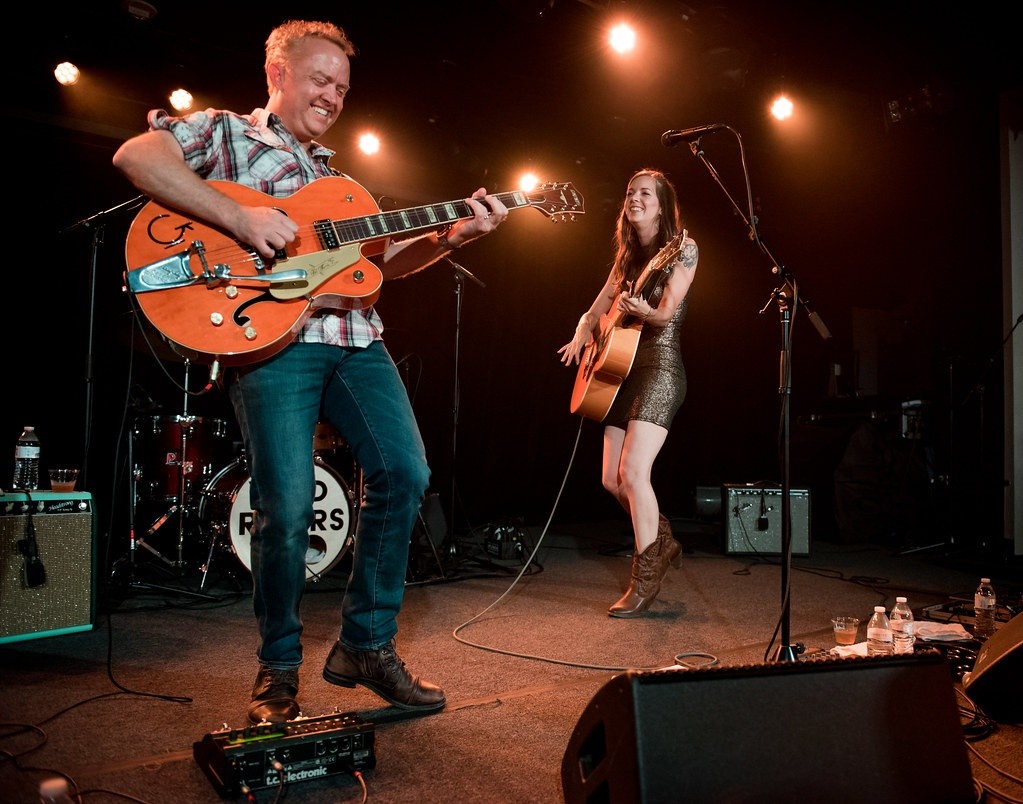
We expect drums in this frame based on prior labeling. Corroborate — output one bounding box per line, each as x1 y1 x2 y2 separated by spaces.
197 455 356 583
141 415 229 507
309 419 351 452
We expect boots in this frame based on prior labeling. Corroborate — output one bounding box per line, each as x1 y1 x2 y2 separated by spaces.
606 535 662 618
658 513 683 583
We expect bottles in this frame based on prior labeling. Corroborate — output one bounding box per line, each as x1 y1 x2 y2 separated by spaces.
893 596 915 655
974 578 996 636
867 606 894 657
12 425 40 491
36 776 76 804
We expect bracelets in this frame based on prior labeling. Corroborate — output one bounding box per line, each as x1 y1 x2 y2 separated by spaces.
436 224 455 252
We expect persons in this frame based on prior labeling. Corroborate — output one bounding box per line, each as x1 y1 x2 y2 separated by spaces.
558 169 699 618
111 19 508 724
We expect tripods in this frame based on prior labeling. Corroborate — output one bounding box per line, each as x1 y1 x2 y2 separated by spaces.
104 359 228 603
417 257 517 581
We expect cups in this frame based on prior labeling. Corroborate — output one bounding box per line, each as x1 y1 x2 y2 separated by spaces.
47 469 81 492
830 616 860 644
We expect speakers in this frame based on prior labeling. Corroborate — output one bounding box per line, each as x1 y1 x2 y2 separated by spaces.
0 489 97 645
560 650 977 804
965 611 1023 726
720 483 812 559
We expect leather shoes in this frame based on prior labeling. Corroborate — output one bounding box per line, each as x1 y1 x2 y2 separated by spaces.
323 637 446 709
247 664 300 726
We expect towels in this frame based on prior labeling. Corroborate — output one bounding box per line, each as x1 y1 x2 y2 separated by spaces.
888 620 974 643
829 641 868 660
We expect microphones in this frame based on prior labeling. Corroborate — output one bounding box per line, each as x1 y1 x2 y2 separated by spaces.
661 123 725 149
395 353 415 367
757 495 768 531
25 516 47 587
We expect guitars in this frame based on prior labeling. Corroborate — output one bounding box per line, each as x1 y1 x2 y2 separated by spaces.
124 175 585 367
569 225 691 421
